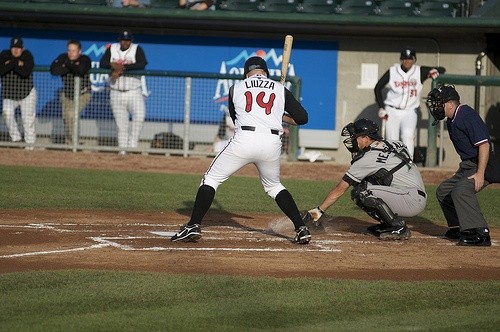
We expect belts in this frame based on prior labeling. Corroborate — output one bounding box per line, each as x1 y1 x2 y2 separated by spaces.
417 190 425 198
241 126 279 135
386 104 418 110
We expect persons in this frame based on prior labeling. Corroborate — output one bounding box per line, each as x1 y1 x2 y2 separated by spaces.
171 57 311 243
113 0 151 9
50 40 91 151
303 119 426 239
178 0 215 10
0 38 37 151
100 29 147 148
426 86 495 246
375 48 445 164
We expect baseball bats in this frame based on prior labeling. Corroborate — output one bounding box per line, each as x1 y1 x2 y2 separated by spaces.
280 35 294 86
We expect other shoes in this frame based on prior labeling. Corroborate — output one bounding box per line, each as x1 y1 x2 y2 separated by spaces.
24 146 34 150
118 150 127 155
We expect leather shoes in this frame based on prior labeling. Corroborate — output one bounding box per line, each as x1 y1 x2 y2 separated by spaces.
458 231 491 246
445 227 460 239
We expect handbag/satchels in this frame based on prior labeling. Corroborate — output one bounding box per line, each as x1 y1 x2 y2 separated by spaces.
477 148 500 182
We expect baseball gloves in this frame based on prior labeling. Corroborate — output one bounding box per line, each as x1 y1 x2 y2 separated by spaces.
298 209 326 234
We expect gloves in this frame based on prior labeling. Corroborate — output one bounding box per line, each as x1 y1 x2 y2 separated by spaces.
302 206 325 223
378 107 388 120
428 68 439 79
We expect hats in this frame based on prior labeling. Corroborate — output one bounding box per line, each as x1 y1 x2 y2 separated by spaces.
10 38 23 47
244 57 269 78
427 85 459 103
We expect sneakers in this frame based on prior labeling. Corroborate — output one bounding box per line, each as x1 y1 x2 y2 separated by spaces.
289 226 311 244
367 224 390 234
379 226 410 240
171 224 202 243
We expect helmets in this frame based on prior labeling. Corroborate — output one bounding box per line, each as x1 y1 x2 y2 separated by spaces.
400 48 416 59
119 29 131 40
354 119 384 141
